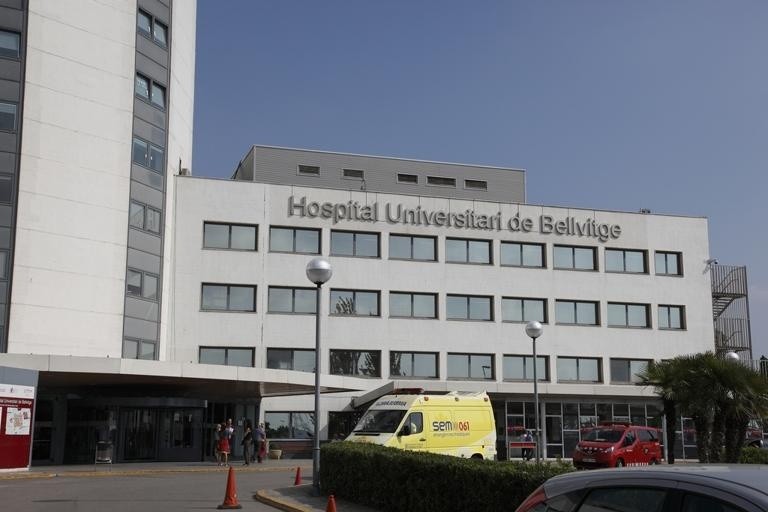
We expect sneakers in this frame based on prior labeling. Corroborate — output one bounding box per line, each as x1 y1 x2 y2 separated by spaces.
218 458 263 468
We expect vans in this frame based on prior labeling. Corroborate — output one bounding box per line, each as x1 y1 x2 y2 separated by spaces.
572 420 662 470
344 387 498 461
503 426 525 457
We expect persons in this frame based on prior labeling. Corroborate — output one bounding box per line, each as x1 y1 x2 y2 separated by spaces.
525 430 534 461
405 416 417 434
212 417 269 469
517 429 531 460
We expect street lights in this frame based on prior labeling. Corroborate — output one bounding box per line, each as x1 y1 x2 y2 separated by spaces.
306 257 333 498
525 320 543 462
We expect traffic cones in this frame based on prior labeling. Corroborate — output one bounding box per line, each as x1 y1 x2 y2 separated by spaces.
326 494 336 512
294 466 303 486
217 466 242 509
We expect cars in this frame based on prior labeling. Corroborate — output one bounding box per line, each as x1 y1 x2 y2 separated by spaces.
515 463 768 512
745 438 768 448
